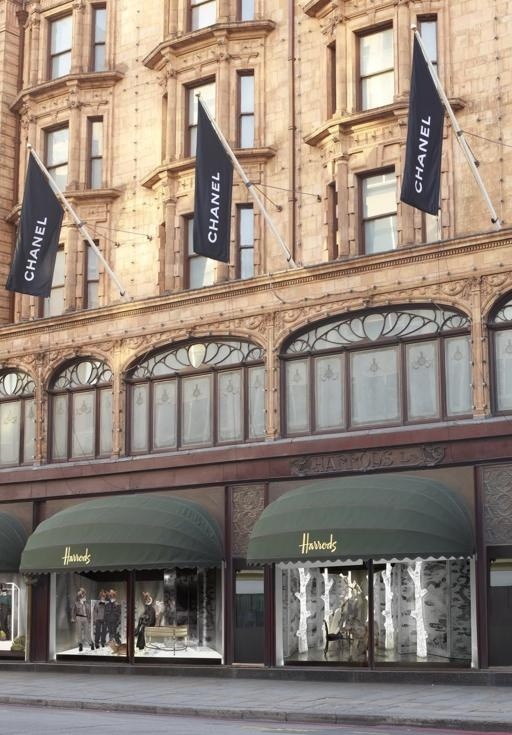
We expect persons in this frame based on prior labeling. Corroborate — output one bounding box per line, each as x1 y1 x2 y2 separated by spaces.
71 588 156 651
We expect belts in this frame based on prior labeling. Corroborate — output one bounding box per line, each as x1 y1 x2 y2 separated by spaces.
76 615 87 617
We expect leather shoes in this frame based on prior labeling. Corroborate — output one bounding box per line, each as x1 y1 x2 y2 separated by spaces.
79 644 105 651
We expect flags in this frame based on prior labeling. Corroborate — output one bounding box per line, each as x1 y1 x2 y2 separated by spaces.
4 149 65 299
192 99 234 262
399 34 444 215
